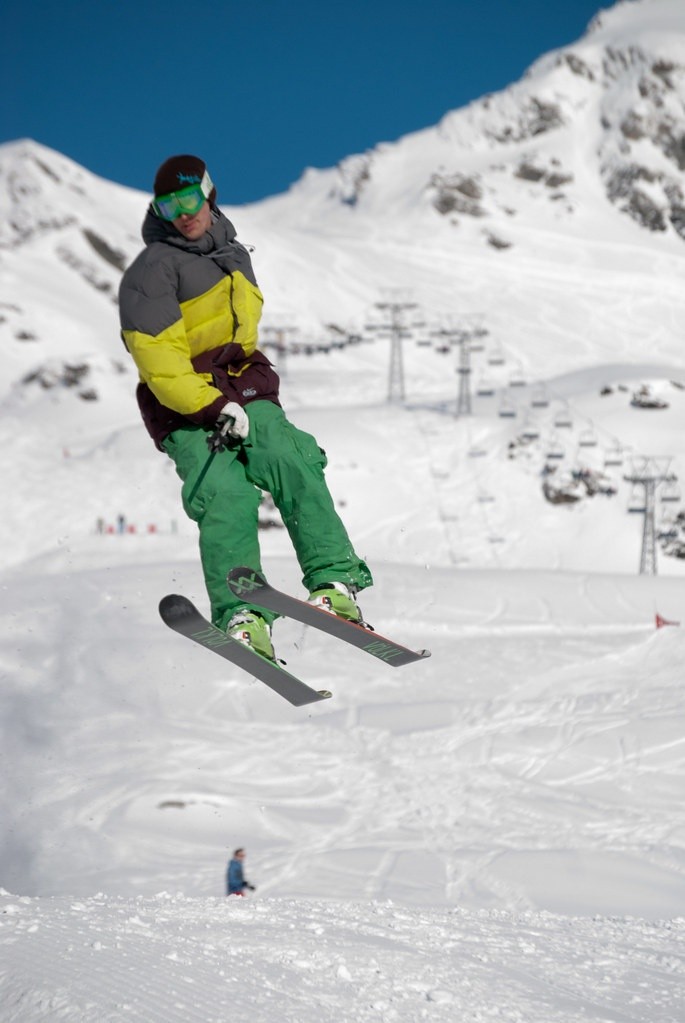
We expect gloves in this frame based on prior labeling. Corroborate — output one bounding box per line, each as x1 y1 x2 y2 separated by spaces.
215 399 250 440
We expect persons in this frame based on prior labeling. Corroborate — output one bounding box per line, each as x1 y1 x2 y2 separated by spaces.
226 848 256 896
119 155 373 668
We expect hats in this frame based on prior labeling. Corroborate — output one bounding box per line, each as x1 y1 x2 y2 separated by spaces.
153 154 216 210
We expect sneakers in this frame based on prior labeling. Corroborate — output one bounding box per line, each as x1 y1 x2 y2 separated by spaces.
229 609 272 655
307 580 358 622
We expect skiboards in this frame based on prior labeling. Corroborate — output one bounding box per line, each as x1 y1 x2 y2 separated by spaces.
159 566 432 707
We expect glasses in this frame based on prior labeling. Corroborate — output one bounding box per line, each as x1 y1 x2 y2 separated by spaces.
153 184 204 222
